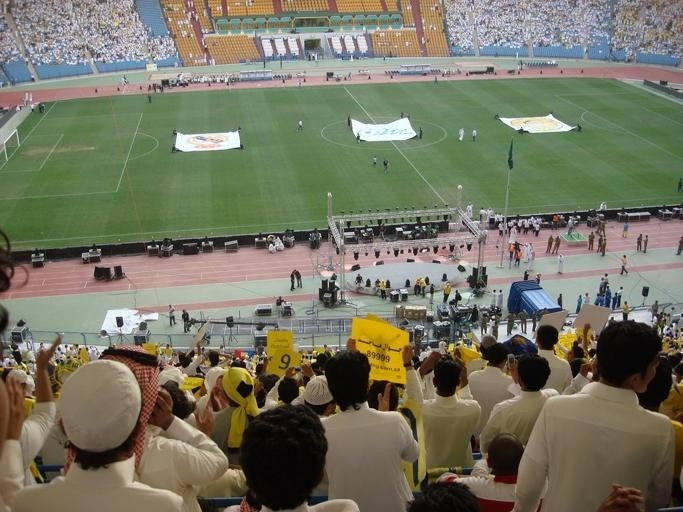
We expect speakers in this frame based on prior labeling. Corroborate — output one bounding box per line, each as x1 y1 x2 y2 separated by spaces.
433 260 440 263
322 279 327 290
643 287 649 296
116 317 123 327
481 266 486 275
329 280 335 290
227 317 233 327
473 267 478 276
407 259 415 262
376 260 384 265
352 264 360 271
114 266 122 277
458 265 466 271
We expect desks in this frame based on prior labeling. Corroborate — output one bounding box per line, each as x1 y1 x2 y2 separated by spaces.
270 235 295 248
346 228 413 243
32 254 45 266
83 249 101 263
225 240 240 251
309 233 322 248
147 239 213 256
615 207 682 222
255 238 267 249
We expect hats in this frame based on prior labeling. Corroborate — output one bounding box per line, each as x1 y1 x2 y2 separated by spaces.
482 335 497 348
5 369 27 384
58 359 141 453
303 375 333 406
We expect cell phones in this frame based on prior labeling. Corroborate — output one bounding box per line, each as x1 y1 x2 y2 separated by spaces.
508 354 515 370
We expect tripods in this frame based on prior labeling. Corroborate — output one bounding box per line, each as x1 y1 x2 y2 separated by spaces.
116 327 129 344
228 327 238 344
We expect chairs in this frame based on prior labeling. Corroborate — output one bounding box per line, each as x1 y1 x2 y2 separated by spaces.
473 452 482 461
1 2 683 65
420 466 475 490
197 496 245 512
308 495 330 505
33 460 67 485
